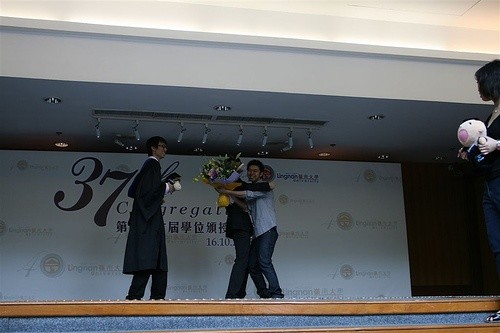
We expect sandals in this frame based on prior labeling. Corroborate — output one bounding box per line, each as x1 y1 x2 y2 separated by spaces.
485 311 500 323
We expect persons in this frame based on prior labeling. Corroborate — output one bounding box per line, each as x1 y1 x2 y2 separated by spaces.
458 59 500 323
122 136 173 300
216 159 284 299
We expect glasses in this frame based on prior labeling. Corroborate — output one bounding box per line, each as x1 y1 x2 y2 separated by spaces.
158 144 166 149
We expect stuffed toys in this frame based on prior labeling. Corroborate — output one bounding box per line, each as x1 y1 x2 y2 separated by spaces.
457 117 493 175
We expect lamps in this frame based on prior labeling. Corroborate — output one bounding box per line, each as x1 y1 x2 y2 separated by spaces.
177 122 184 142
95 118 101 138
202 123 208 144
262 127 269 147
287 127 294 149
306 128 314 149
132 120 140 141
236 124 243 146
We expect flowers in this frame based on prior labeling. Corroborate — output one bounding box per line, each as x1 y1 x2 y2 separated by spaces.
192 151 245 208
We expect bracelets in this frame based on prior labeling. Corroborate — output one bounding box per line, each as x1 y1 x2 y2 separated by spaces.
496 140 500 150
